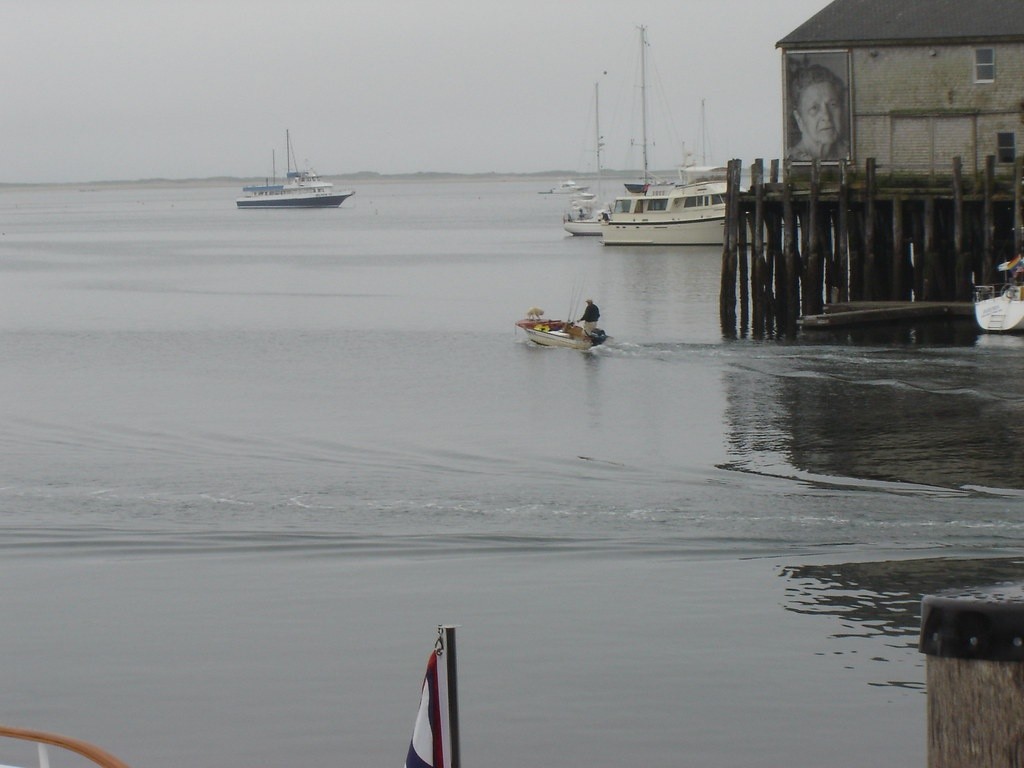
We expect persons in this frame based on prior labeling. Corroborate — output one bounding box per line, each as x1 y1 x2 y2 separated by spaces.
788 64 850 161
577 300 600 336
601 212 610 222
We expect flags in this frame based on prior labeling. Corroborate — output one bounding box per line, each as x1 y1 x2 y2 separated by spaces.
403 649 443 768
1000 254 1024 278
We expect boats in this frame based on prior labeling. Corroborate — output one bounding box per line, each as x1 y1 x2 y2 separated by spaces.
515 307 608 351
536 180 592 194
564 211 603 236
603 22 769 246
236 129 356 208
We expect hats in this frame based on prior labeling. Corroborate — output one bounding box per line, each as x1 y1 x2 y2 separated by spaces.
586 300 592 302
